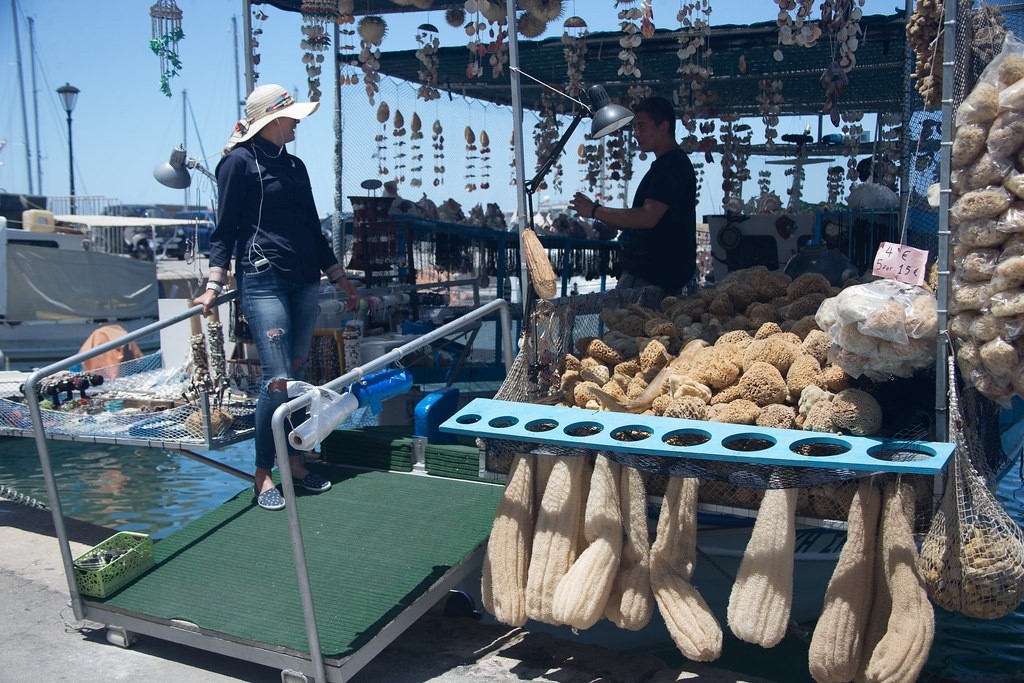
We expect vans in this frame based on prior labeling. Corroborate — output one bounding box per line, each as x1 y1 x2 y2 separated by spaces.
96 206 186 260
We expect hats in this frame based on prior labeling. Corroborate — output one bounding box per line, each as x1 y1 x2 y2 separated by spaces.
224 84 320 156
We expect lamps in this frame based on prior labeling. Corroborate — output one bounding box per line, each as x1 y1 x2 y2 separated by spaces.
526 84 636 195
152 143 218 190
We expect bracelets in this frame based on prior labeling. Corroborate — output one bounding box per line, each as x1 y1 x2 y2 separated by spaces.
205 283 222 293
591 200 602 219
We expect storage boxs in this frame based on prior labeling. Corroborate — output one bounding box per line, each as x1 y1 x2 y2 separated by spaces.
72 532 155 598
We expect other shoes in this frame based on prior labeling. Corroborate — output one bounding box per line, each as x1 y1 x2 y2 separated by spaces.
292 472 332 491
253 483 285 510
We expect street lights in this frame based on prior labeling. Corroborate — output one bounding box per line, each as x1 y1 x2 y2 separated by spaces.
56 82 81 214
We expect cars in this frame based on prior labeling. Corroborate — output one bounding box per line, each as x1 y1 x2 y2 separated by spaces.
172 210 238 258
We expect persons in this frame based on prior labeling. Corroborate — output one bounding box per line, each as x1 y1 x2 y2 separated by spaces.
568 98 696 289
193 84 360 510
